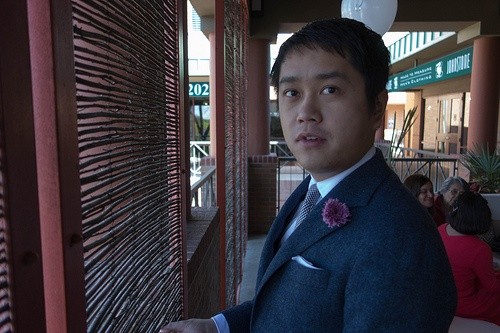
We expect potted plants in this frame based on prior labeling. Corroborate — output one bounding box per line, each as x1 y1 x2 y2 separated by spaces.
457 141 500 220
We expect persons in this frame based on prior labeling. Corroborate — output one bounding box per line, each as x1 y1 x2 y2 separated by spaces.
434 176 470 223
404 174 434 215
159 17 458 333
437 191 500 326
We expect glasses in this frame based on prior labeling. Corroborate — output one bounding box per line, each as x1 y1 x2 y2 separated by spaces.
447 190 463 196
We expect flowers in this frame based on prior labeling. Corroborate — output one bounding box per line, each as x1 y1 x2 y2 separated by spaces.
321 198 351 228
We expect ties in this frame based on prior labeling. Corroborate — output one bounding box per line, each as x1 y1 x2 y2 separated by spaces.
294 184 320 231
